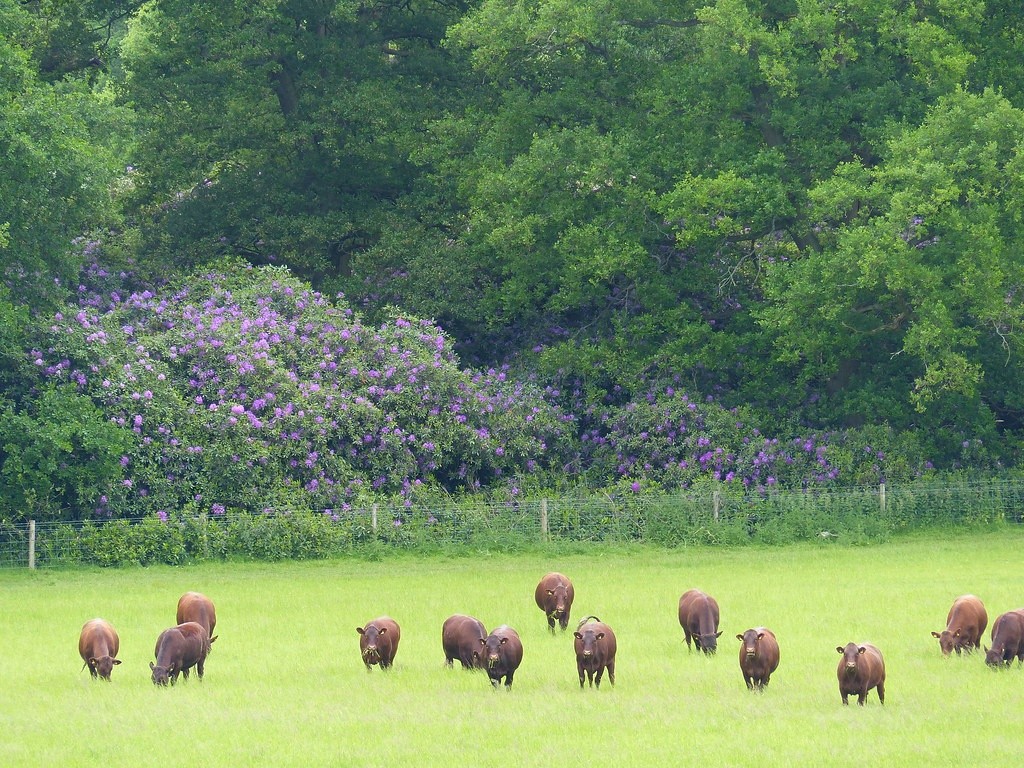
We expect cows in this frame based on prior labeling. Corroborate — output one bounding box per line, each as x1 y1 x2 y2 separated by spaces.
930 595 988 660
736 627 779 694
79 618 122 682
442 614 487 669
983 608 1024 672
149 622 211 689
535 572 575 635
835 642 886 706
177 592 218 644
679 588 724 658
477 626 523 692
357 616 400 673
574 615 617 689
